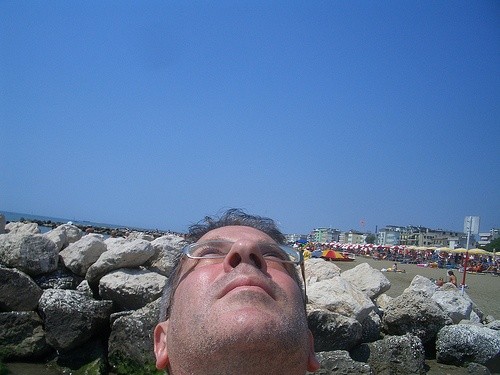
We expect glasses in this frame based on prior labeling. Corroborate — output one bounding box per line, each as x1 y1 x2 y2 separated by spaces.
165 241 309 318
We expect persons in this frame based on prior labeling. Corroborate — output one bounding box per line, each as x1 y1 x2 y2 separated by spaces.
447 271 457 287
152 207 321 375
373 250 500 277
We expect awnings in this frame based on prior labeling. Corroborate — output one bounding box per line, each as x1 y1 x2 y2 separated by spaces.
294 239 500 261
